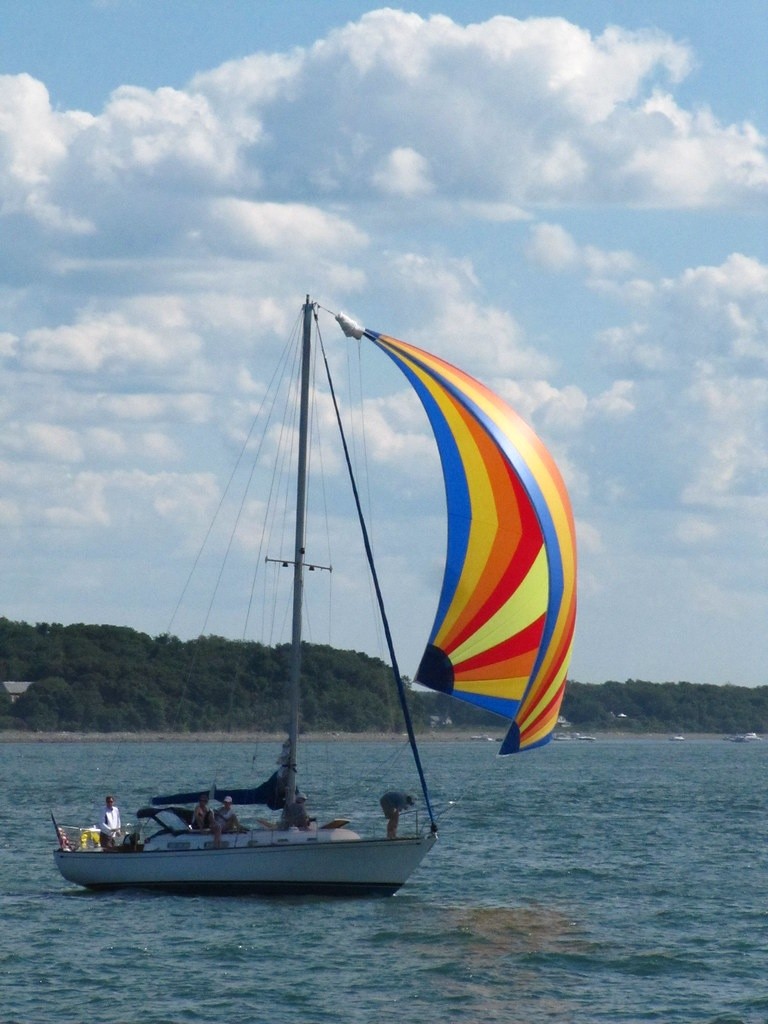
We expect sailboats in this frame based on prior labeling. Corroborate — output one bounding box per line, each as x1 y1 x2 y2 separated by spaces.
46 295 579 900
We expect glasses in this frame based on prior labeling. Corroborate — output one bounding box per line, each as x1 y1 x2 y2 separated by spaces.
107 801 113 804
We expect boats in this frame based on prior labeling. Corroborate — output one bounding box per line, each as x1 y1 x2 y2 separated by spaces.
472 731 595 743
670 735 686 741
723 733 763 743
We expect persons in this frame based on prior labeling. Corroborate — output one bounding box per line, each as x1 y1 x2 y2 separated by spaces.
380 791 418 838
287 794 310 829
216 796 245 847
192 795 215 830
101 796 122 851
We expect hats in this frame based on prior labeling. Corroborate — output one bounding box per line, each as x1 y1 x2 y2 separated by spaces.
224 796 232 803
199 795 208 802
297 793 308 800
410 794 418 804
